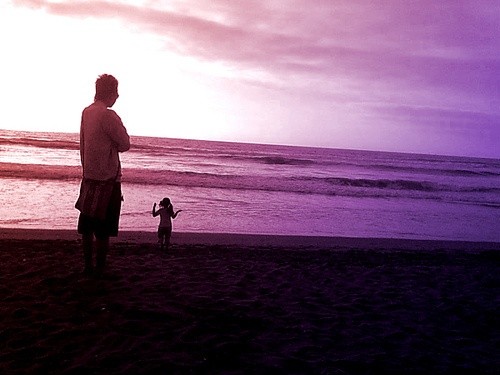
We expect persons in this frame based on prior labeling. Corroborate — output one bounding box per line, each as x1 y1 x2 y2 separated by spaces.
75 75 130 280
152 197 183 245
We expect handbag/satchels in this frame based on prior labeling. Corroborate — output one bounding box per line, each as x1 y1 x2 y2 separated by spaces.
75 178 112 219
